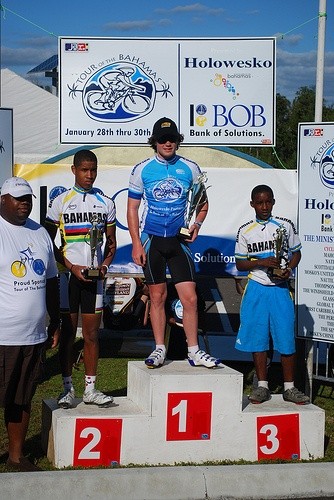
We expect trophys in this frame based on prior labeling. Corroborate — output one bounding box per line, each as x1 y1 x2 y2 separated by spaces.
178 171 207 239
83 214 104 278
269 224 288 276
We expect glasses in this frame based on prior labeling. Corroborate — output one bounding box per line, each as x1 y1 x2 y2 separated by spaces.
158 136 177 144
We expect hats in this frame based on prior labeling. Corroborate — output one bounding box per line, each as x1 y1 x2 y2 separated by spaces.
1 176 36 199
153 117 177 136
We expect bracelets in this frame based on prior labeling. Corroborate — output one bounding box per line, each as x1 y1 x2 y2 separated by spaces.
194 221 202 227
70 265 75 271
102 264 108 272
287 267 292 273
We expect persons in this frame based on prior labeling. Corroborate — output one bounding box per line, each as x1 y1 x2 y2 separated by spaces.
234 185 310 405
0 176 61 472
44 150 117 408
127 116 221 369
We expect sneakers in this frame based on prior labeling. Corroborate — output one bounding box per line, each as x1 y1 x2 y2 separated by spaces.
145 348 168 368
6 457 42 471
57 392 75 409
82 389 113 408
248 386 271 403
283 387 310 405
188 349 221 368
